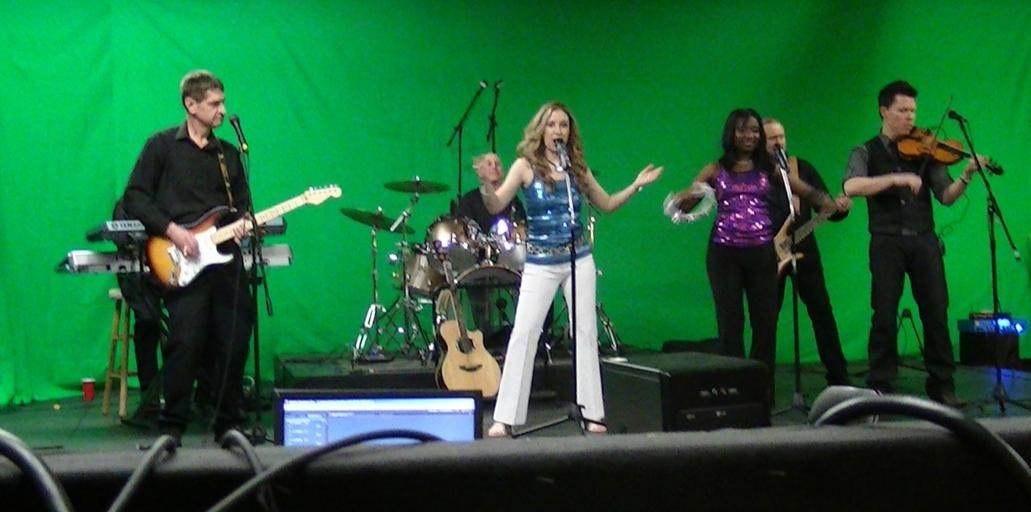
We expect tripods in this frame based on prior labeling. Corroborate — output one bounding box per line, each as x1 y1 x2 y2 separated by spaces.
771 224 812 420
363 241 432 367
350 251 406 367
952 220 1031 412
509 231 626 439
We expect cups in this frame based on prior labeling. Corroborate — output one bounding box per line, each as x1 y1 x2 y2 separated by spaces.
82 379 96 400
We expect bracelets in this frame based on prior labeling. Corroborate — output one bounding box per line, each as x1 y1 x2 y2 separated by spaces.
960 176 967 184
634 182 642 192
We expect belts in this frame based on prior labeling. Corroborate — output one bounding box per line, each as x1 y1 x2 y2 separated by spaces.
893 226 920 236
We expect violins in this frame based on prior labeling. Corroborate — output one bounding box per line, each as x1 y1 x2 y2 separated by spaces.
897 127 1004 175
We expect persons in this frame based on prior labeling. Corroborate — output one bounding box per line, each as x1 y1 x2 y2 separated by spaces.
762 118 868 408
459 153 554 342
126 70 261 448
474 101 664 438
676 107 835 395
115 187 188 431
841 81 984 410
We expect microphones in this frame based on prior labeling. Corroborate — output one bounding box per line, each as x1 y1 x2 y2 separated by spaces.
391 207 413 233
228 113 249 153
480 80 488 89
771 142 791 175
554 139 570 168
948 111 966 121
494 80 506 90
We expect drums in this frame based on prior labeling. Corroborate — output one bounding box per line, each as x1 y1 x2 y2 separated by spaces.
425 215 480 278
486 220 529 270
452 260 521 287
401 243 445 300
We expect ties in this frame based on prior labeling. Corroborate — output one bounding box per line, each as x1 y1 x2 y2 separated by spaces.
888 140 900 164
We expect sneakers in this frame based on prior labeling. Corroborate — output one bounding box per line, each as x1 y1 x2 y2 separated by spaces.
581 419 607 433
488 422 512 437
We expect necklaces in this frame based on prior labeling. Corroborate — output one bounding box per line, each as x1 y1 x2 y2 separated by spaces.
731 158 753 166
543 153 566 172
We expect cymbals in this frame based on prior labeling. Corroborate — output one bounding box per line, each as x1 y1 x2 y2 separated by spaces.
344 206 415 234
384 178 451 195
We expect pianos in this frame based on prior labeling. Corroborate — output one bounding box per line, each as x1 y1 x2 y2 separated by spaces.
53 240 294 273
84 215 286 244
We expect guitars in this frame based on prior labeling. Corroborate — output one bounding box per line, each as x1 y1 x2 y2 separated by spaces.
433 239 502 399
773 195 847 282
145 183 342 288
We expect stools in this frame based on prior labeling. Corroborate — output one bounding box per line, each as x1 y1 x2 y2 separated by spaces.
99 287 165 418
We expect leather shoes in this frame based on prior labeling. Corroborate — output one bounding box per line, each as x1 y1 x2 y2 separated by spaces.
139 431 183 449
216 424 265 446
930 386 968 408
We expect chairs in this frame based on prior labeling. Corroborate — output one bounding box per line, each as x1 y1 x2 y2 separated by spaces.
808 383 882 424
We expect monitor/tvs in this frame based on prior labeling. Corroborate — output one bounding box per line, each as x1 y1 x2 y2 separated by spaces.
274 388 482 448
